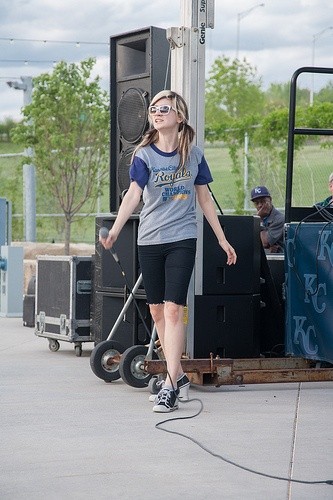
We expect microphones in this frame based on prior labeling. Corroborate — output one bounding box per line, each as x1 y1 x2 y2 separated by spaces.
99 227 120 265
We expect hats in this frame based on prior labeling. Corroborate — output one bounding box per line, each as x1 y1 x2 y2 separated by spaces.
250 186 271 202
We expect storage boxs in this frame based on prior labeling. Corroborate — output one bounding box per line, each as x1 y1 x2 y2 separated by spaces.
35 255 92 342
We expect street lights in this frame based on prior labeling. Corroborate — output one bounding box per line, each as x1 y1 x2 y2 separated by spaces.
236 3 266 63
310 27 333 107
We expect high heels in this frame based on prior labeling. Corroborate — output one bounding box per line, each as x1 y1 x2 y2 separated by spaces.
153 387 180 413
148 371 191 403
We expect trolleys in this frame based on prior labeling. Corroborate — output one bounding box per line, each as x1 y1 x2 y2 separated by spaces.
89 273 162 388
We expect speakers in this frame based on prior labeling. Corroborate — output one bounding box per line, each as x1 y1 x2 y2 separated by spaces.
90 25 285 362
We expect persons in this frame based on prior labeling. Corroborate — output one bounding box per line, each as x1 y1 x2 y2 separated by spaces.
98 89 237 413
313 172 333 207
250 186 288 253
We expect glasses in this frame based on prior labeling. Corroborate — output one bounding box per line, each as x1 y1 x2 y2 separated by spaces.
148 105 176 115
